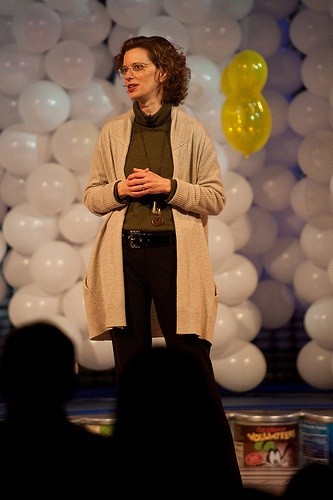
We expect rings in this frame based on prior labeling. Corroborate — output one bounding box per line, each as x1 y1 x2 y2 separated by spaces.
142 185 144 190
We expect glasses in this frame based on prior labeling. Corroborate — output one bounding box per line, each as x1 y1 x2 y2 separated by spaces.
117 61 158 77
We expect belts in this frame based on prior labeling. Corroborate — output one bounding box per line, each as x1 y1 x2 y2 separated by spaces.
121 230 176 249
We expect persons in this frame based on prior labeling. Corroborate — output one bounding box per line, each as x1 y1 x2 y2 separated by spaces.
0 321 333 499
82 36 225 366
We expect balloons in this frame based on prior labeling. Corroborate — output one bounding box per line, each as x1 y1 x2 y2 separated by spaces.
180 50 333 391
1 0 118 371
107 0 333 64
